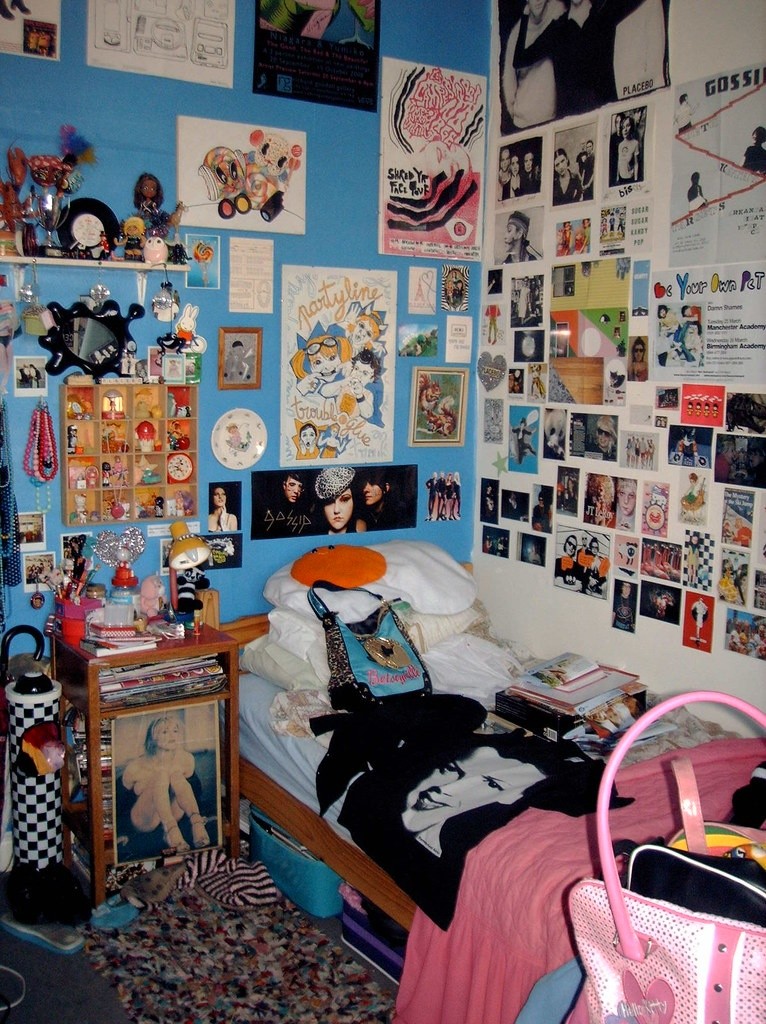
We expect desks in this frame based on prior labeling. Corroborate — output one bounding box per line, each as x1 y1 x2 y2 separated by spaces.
46 612 241 907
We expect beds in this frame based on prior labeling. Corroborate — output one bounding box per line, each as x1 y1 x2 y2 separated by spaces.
201 562 764 1022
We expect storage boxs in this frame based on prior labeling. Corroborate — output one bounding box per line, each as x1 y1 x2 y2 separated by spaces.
246 804 344 918
340 885 410 986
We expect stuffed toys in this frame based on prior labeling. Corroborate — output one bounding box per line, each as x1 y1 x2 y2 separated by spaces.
176 559 210 613
138 576 166 617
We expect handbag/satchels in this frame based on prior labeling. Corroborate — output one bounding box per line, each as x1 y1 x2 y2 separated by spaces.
567 692 766 1024
308 579 432 714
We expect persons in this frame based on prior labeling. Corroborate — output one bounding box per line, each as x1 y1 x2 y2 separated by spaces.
114 217 146 261
283 466 405 538
134 173 170 225
115 713 210 853
98 231 109 261
208 487 237 530
425 471 461 521
25 528 87 585
482 0 766 662
19 363 42 388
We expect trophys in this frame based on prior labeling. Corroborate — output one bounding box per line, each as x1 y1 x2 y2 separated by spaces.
29 192 70 258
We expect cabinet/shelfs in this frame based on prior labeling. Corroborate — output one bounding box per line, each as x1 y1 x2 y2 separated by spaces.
60 381 199 526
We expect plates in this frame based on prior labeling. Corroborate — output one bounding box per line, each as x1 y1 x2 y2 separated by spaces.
211 408 268 471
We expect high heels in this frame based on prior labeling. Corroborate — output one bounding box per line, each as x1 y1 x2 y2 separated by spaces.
189 813 209 847
163 825 190 852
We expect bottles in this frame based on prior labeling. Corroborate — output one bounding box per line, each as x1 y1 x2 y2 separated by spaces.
104 587 140 626
86 583 107 606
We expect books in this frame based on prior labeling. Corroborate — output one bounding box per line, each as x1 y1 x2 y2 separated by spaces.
79 634 162 658
511 653 644 717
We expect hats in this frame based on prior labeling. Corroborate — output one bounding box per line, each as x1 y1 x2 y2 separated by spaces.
315 466 356 501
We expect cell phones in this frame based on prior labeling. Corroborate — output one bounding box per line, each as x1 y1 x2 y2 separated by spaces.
0 909 86 954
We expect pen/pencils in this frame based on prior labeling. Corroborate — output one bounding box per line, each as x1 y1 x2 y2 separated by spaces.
45 558 100 599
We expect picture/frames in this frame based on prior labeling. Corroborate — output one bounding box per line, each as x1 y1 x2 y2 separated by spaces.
218 327 262 389
408 366 470 447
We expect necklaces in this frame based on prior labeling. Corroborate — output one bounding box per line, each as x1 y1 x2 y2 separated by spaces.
22 398 59 514
0 403 17 635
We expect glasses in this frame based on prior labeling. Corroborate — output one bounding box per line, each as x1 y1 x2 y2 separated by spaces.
539 499 544 501
582 538 587 540
285 482 305 492
591 546 598 549
597 428 613 437
635 348 645 352
567 541 576 550
